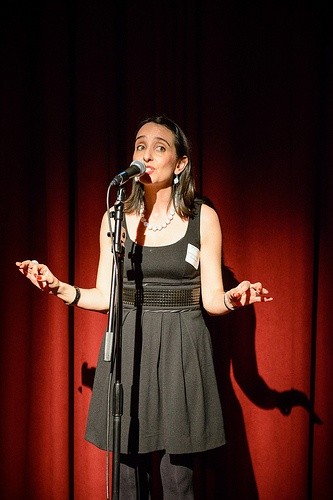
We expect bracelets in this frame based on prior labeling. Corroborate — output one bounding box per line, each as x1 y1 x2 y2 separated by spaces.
224 292 236 311
65 284 80 306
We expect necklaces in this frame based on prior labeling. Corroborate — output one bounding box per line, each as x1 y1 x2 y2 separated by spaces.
136 199 178 232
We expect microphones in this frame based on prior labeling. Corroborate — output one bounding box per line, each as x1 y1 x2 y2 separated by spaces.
112 160 146 185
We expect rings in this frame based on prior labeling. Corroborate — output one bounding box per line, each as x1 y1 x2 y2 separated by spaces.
32 259 39 264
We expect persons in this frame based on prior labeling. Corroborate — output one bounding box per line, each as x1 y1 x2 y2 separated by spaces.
15 115 274 500
